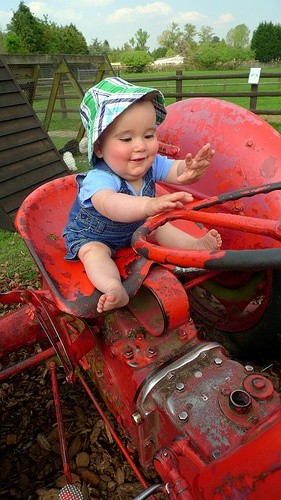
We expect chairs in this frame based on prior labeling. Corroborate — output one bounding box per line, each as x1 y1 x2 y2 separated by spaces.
15 170 221 318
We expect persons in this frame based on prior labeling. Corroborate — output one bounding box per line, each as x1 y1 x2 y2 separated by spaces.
61 77 222 313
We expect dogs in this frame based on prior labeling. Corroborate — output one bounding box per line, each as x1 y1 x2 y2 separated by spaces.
79 135 88 154
62 151 77 170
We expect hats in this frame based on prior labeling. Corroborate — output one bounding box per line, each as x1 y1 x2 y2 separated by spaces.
80 77 167 168
